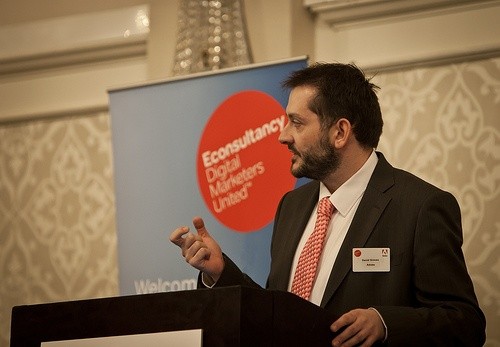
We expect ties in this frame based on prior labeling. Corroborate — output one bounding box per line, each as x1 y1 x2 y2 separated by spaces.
288 193 335 302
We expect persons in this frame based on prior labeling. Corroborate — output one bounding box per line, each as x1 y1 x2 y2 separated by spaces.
170 58 488 347
